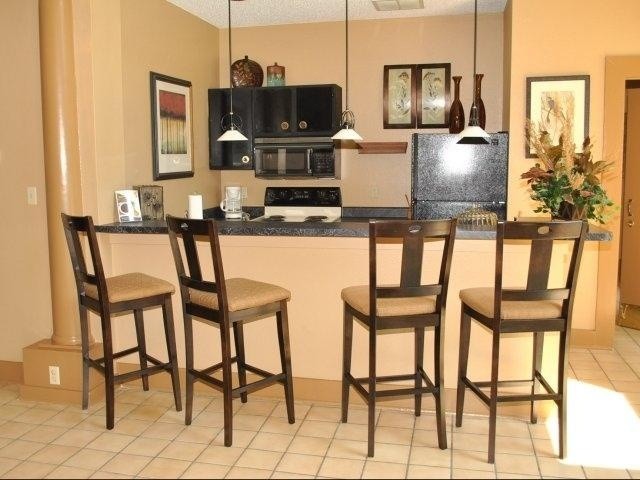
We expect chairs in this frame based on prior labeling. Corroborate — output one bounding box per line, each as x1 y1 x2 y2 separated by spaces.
164 215 296 447
457 218 589 462
63 212 183 431
341 217 449 459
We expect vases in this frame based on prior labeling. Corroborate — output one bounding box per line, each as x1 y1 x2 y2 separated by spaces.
449 76 466 133
549 198 589 221
468 74 487 132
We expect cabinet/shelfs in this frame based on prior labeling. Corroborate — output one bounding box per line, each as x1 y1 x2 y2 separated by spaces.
254 84 343 138
330 0 362 141
208 87 254 171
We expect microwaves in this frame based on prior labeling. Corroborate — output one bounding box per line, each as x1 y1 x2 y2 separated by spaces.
250 215 341 223
253 144 336 179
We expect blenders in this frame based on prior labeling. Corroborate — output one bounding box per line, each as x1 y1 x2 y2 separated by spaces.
219 185 243 219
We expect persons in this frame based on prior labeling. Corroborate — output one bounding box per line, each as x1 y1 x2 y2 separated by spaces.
434 78 444 107
394 73 411 117
422 72 435 109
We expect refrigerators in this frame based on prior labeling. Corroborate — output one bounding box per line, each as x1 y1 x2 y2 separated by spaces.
411 131 508 222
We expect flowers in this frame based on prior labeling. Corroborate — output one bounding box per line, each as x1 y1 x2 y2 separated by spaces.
519 119 619 224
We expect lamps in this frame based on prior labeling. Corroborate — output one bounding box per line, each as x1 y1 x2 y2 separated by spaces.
458 1 491 138
216 1 249 142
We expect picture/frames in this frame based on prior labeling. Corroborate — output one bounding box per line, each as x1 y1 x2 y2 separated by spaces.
526 75 592 158
417 63 452 128
384 64 417 129
150 71 195 181
